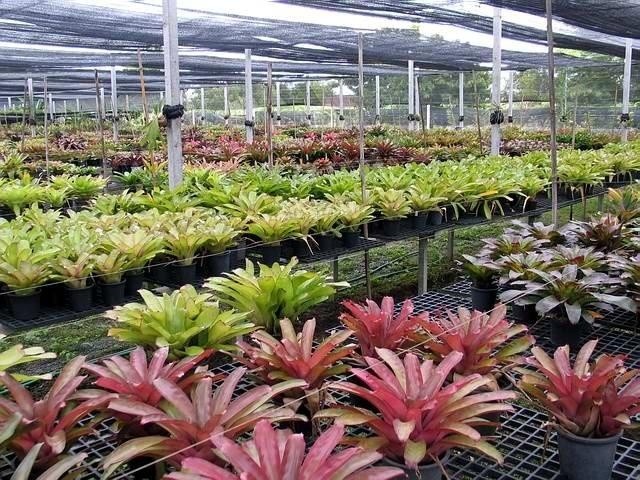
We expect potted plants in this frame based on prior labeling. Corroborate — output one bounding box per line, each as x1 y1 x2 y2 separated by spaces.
0 142 640 479
